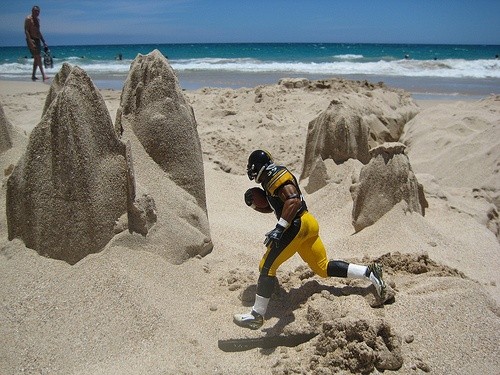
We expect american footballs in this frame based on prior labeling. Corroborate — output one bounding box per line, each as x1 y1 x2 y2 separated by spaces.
250 186 269 209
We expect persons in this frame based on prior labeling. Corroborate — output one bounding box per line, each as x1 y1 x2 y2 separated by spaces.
233 149 386 330
24 6 51 81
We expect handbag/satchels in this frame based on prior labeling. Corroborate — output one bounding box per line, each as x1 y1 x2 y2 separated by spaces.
41 49 52 68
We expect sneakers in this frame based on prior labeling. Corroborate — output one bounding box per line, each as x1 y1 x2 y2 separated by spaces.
233 306 263 329
363 263 386 298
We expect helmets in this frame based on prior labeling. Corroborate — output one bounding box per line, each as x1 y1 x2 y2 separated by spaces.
247 150 278 184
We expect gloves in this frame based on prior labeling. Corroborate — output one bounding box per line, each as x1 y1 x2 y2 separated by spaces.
263 217 290 249
